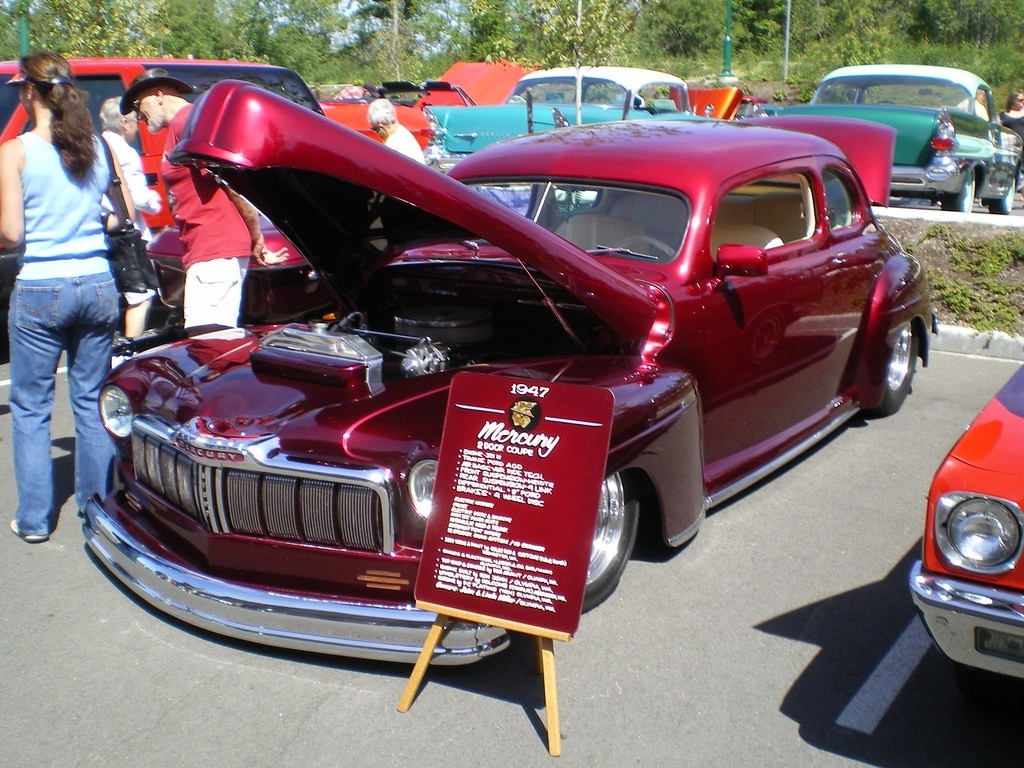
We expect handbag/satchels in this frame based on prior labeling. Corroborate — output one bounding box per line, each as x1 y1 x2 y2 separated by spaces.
96 134 160 293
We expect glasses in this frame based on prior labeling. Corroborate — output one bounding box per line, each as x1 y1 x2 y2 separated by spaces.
372 124 390 131
1017 99 1024 102
132 90 156 113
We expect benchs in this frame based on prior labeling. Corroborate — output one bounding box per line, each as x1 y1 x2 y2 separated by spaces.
611 190 808 260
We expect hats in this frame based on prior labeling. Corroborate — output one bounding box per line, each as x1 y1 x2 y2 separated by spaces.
120 69 196 115
4 73 53 90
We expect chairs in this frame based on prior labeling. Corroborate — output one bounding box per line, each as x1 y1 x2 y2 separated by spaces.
710 223 784 265
553 212 650 260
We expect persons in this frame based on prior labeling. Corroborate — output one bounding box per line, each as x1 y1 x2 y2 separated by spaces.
0 50 139 540
97 70 426 360
958 90 1024 207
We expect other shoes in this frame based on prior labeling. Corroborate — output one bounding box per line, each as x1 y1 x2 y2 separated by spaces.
11 520 49 543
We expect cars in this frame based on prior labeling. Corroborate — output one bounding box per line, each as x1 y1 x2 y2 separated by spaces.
906 361 1024 684
776 62 1022 216
80 80 939 668
1 57 782 353
